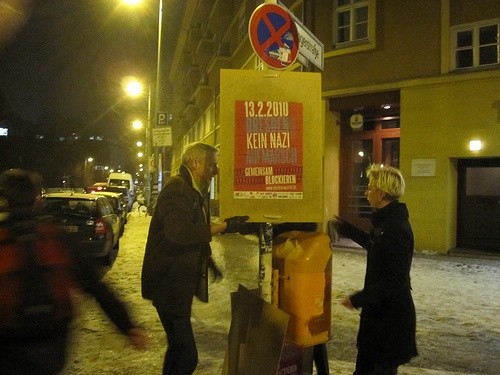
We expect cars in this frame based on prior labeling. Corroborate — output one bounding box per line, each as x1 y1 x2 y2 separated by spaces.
88 172 137 226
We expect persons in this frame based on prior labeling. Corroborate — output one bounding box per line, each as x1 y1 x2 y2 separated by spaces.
139 143 255 375
330 163 419 375
0 168 149 374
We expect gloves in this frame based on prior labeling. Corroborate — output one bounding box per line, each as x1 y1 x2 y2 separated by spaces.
221 215 257 233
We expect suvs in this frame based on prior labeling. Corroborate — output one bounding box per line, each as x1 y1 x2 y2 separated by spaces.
33 191 121 265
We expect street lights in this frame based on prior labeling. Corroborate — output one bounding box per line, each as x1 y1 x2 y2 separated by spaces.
84 158 114 188
124 78 154 217
